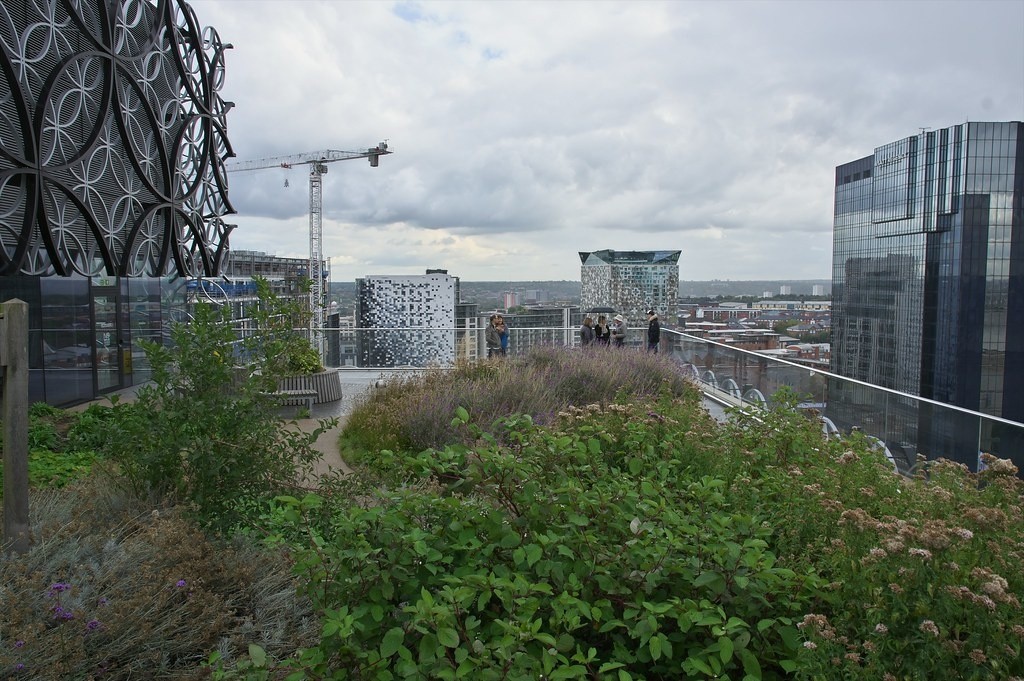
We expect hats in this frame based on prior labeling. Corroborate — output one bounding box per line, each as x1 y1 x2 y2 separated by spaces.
645 310 654 315
615 314 623 322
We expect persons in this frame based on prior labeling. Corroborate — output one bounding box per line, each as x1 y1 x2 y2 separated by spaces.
611 315 627 348
579 318 594 348
594 316 610 347
485 314 502 361
645 310 660 354
495 315 510 357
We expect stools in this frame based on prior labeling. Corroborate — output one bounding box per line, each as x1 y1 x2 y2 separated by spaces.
259 388 318 421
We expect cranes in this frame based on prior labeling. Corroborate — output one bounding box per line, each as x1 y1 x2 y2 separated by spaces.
212 139 395 367
